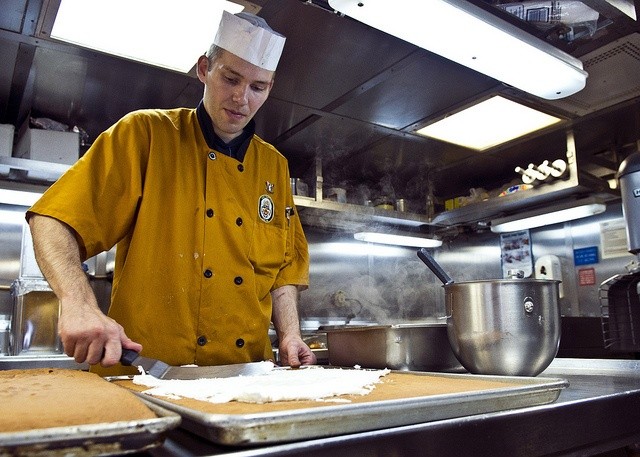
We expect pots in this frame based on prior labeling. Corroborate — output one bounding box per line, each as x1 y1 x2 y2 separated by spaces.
440 278 563 375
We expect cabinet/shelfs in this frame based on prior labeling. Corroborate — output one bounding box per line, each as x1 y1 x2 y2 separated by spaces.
430 130 579 224
0 107 430 227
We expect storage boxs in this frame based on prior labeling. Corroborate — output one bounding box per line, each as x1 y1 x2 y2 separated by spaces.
0 124 16 158
14 114 79 166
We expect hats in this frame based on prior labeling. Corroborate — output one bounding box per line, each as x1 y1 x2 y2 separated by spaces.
213 8 287 72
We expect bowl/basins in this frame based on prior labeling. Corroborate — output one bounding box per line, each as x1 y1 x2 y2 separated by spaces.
318 322 467 373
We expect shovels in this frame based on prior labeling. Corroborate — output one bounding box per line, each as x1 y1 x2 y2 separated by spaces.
102 345 276 380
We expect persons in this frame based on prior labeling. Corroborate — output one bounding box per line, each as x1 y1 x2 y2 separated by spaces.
29 12 319 365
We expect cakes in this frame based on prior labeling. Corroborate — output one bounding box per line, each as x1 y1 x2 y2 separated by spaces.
106 372 526 417
0 368 158 433
